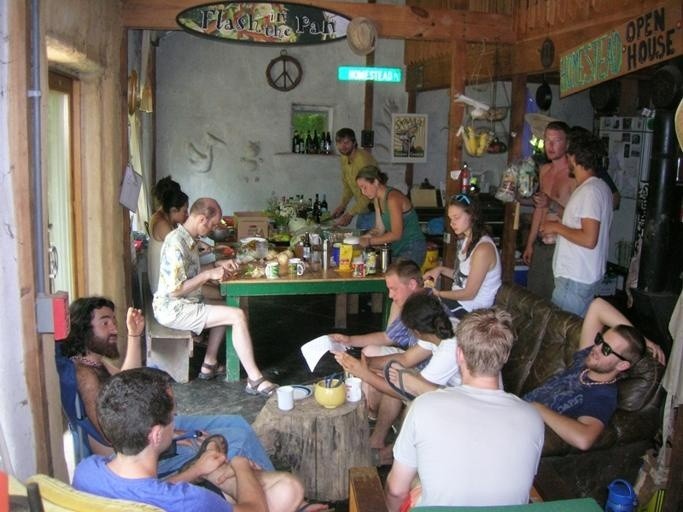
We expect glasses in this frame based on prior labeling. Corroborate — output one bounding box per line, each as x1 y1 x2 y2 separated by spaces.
452 195 470 204
595 332 628 360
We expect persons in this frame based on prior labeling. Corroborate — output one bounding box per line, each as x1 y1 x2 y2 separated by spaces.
371 298 665 465
355 165 427 269
329 255 544 511
72 367 305 512
539 137 614 319
329 127 378 230
146 188 232 347
68 296 335 512
568 125 621 211
523 122 576 300
151 197 280 398
148 177 180 213
387 194 502 331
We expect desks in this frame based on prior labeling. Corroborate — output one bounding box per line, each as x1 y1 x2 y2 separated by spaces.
213 259 392 385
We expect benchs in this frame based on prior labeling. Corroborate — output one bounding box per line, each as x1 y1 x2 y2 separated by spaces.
141 269 194 384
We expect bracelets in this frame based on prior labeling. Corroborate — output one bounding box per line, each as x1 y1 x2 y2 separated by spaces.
127 334 141 337
368 238 371 246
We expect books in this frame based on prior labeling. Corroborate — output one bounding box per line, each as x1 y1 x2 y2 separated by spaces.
119 166 144 213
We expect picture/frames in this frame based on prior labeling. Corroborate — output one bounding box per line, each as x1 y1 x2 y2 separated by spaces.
390 112 428 164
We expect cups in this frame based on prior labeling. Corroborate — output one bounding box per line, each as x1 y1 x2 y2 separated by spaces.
265 226 392 279
276 377 362 411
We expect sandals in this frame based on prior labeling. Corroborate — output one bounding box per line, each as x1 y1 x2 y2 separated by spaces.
178 434 228 484
198 361 227 380
245 377 279 397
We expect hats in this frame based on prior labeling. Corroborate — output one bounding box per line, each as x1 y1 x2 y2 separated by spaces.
348 17 378 56
525 113 560 138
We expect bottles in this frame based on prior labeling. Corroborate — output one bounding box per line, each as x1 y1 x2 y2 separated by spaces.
605 479 639 512
461 164 471 196
293 130 331 155
273 193 328 224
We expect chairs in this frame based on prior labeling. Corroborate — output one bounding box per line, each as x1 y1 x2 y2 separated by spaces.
346 462 608 512
1 466 170 511
53 340 202 456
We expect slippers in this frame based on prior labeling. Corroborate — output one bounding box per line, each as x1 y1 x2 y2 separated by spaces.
384 360 417 400
298 502 335 512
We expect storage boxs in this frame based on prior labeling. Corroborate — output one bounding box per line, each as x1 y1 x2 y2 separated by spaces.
232 209 269 245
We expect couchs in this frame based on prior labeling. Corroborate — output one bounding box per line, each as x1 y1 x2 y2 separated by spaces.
518 308 668 503
440 242 557 397
